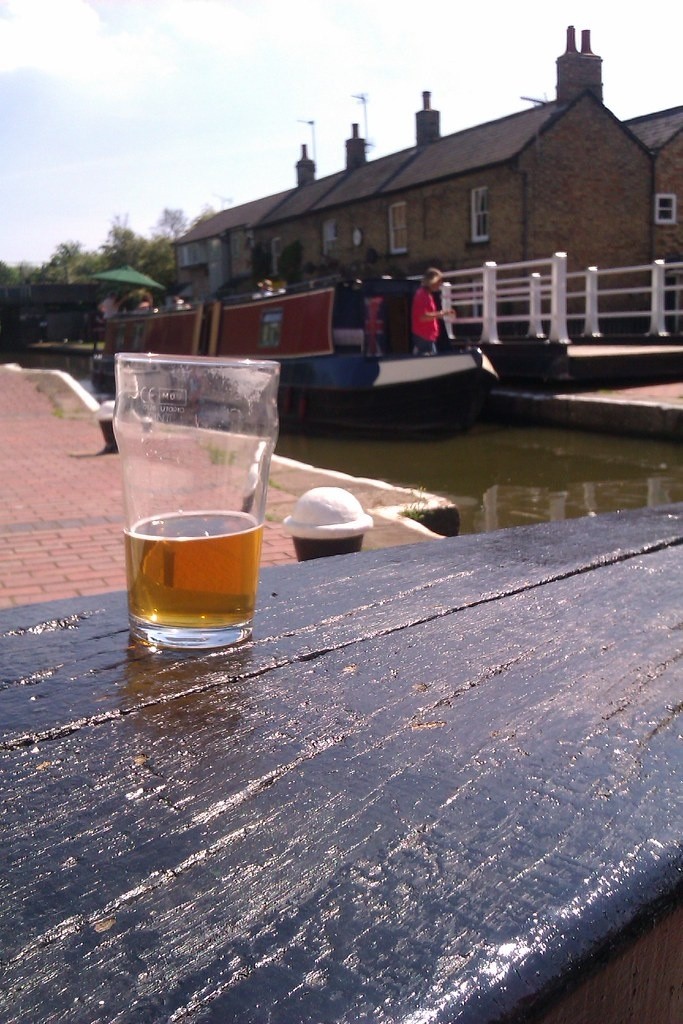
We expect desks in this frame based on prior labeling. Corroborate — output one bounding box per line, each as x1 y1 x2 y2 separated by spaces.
0 505 682 1024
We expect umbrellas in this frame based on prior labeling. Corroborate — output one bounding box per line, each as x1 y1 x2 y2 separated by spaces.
90 266 166 290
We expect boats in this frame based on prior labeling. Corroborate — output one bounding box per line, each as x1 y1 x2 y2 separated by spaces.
87 279 496 441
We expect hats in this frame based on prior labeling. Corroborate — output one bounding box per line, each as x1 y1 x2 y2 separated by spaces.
259 279 274 292
421 267 443 288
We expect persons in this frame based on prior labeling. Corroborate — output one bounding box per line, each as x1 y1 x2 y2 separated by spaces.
409 268 454 355
97 285 156 316
252 279 286 301
172 295 192 311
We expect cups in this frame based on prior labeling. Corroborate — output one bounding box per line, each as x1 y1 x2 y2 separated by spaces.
111 352 281 649
